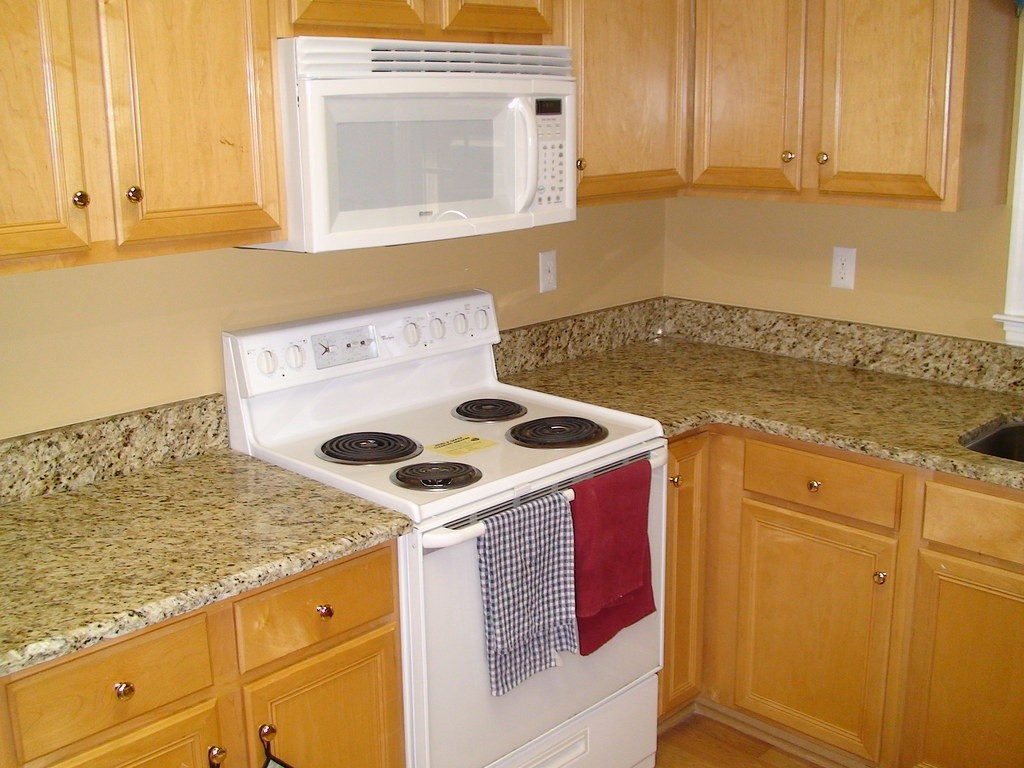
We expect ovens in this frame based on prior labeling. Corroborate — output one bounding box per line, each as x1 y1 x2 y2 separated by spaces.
400 442 667 768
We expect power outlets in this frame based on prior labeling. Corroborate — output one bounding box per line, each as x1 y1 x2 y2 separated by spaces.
830 246 857 290
539 250 557 294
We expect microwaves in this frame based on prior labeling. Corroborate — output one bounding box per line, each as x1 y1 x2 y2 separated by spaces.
277 36 583 252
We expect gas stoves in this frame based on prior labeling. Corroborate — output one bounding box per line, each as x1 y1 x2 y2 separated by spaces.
218 286 663 522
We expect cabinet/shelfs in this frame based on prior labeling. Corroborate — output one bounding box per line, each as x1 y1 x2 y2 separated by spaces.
271 0 553 46
681 0 970 213
0 0 292 275
656 428 713 737
700 420 910 767
1 538 407 768
891 465 1023 768
543 0 691 206
